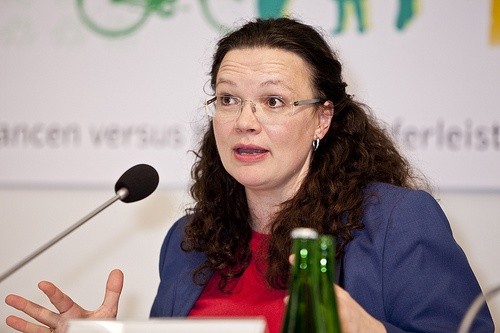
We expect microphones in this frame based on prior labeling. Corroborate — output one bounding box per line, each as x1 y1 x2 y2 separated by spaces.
0 164 159 284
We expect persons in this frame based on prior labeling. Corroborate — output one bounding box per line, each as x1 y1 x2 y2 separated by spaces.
5 16 495 333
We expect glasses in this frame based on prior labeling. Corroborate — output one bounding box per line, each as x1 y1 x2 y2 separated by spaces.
204 96 326 120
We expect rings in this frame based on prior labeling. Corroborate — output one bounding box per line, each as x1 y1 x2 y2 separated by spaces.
50 329 54 333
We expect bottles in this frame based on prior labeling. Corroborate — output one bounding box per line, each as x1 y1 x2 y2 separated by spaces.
281 227 340 333
